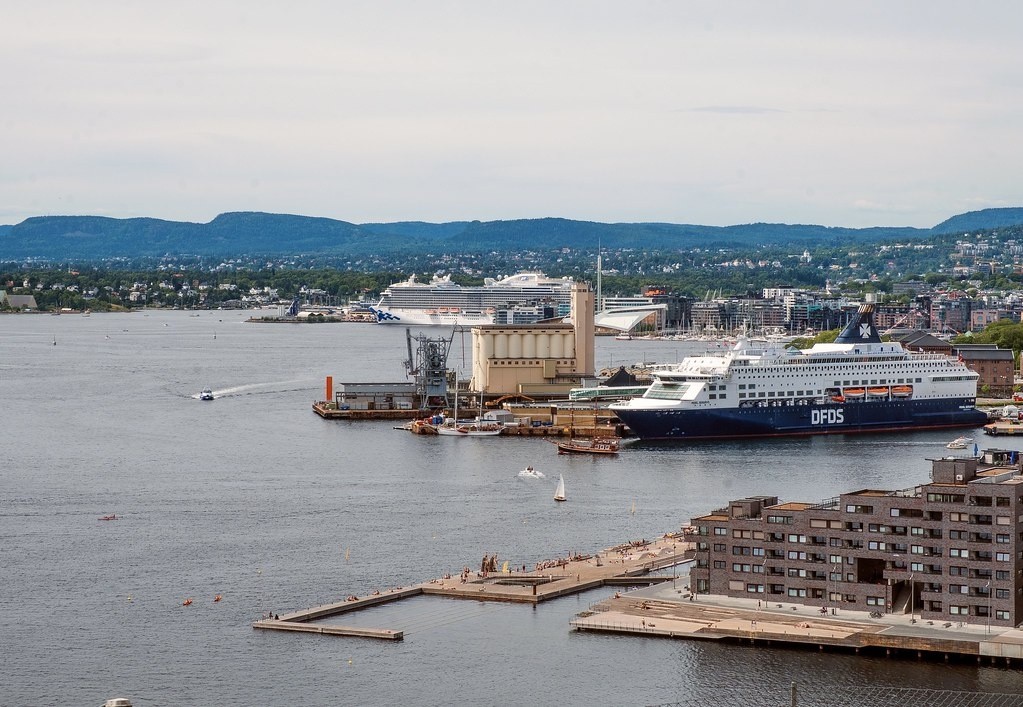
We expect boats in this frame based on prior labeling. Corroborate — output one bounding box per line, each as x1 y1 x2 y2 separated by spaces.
199 386 213 400
519 466 544 479
891 386 912 396
427 309 438 315
844 389 864 398
82 309 90 317
438 307 449 313
486 308 497 314
52 310 60 315
831 396 846 403
947 435 974 450
450 307 459 313
867 387 889 397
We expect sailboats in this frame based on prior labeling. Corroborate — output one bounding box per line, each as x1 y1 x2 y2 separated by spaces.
615 312 783 344
403 363 506 437
556 394 623 454
553 473 567 502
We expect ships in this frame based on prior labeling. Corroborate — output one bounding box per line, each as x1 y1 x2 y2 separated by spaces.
369 270 575 325
605 302 988 446
